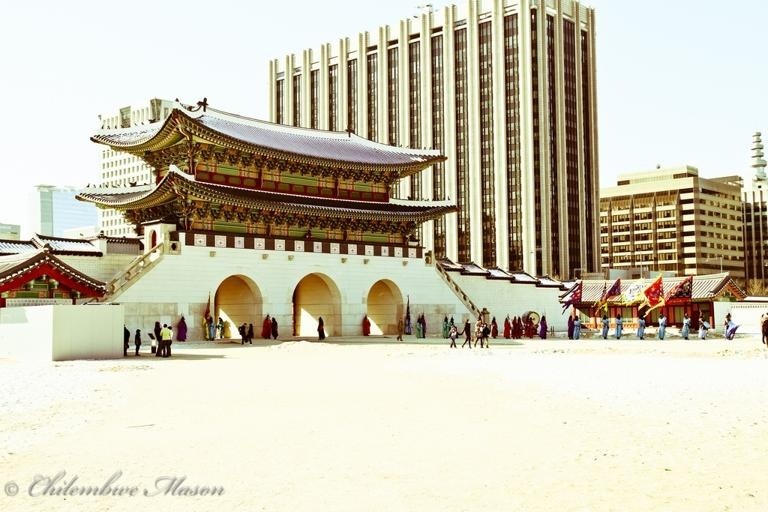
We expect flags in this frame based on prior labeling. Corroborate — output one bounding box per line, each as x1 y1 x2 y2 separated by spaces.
557 272 692 318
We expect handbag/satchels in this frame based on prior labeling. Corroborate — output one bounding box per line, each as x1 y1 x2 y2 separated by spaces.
477 331 484 337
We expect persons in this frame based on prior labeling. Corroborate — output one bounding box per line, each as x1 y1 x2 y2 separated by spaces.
396 312 546 350
364 315 371 335
316 314 327 341
204 314 278 345
124 316 187 357
567 314 768 347
